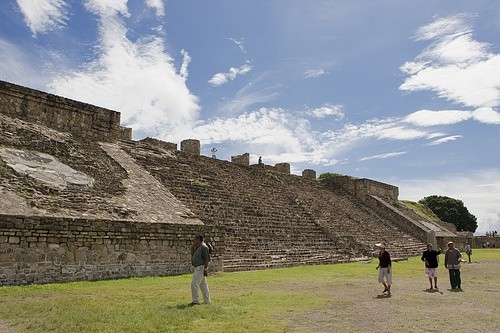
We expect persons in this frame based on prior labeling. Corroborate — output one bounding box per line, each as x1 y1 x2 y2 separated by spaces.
259 156 264 168
375 241 392 297
465 243 472 263
211 148 217 159
188 235 211 305
421 243 441 289
445 242 463 291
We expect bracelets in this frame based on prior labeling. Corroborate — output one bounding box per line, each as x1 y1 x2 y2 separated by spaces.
204 269 208 270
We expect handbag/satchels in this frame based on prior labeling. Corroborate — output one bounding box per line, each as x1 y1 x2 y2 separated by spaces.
206 243 213 254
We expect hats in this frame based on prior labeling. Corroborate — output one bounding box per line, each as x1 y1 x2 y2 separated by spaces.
375 242 386 249
